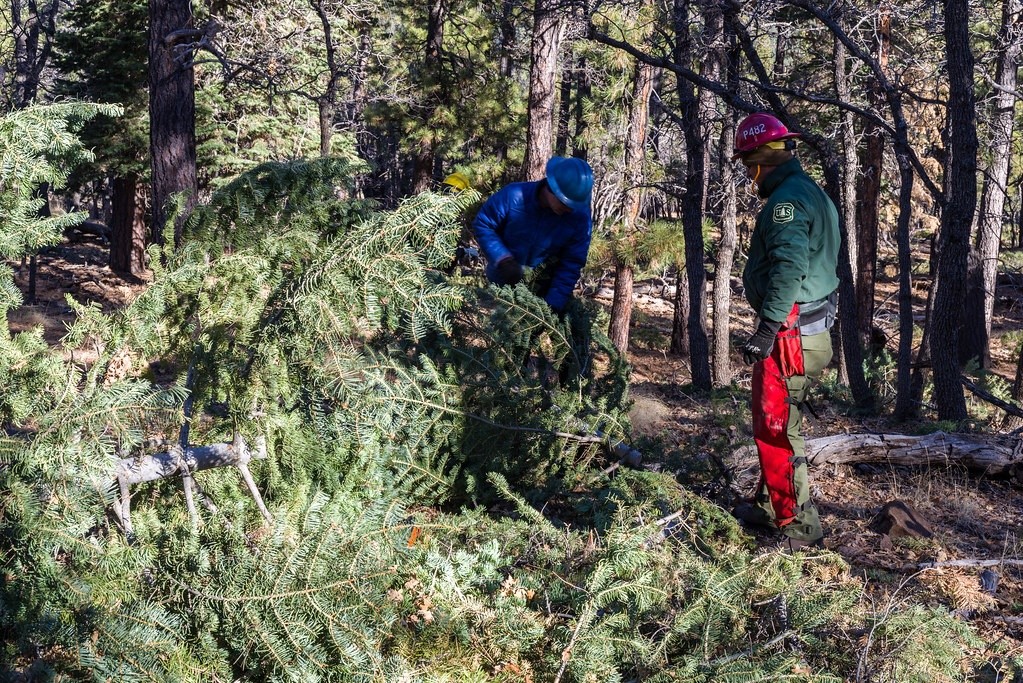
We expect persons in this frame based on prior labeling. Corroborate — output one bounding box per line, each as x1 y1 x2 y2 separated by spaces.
730 113 840 563
412 172 480 391
471 155 594 423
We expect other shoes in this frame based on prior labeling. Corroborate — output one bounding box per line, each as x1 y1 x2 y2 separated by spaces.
731 504 825 554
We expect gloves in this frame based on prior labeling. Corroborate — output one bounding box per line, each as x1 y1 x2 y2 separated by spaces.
740 323 775 367
498 256 521 282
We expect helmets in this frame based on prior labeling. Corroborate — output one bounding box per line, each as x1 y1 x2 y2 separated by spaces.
442 173 471 191
545 156 594 213
733 112 800 162
